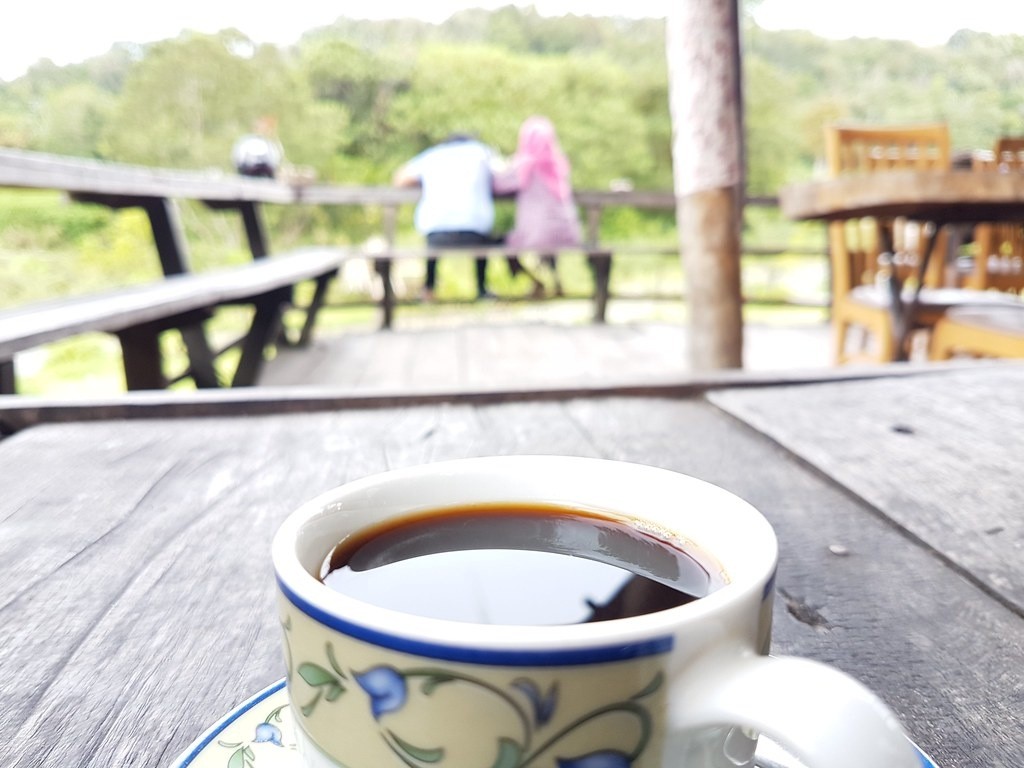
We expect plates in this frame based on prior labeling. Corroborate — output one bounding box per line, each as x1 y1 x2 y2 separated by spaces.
169 679 938 768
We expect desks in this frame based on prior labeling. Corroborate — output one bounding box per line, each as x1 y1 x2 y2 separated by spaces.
0 365 1024 768
783 171 1024 362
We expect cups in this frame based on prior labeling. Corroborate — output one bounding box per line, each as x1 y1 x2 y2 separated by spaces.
269 455 921 768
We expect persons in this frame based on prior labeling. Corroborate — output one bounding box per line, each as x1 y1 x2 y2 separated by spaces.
391 116 586 300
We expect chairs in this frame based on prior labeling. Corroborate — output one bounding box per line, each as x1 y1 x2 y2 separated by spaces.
825 126 1024 362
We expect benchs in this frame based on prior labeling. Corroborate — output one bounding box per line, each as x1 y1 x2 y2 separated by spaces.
0 235 827 395
